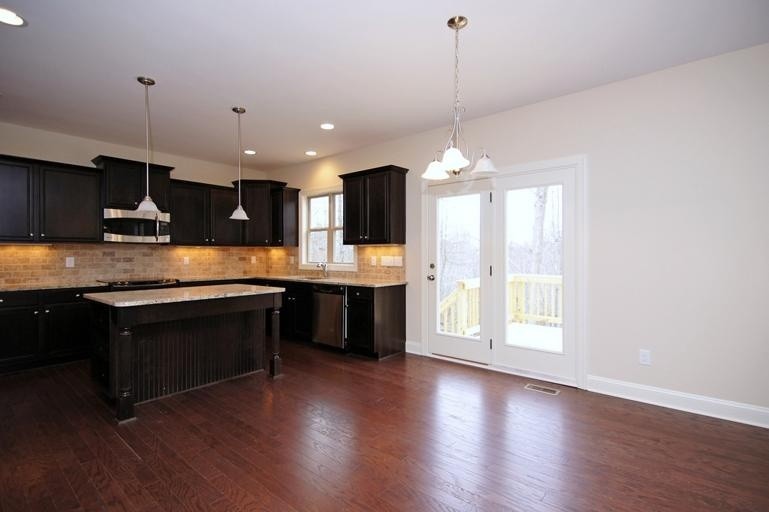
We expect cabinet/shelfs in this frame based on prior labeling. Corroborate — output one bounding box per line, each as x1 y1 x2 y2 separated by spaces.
1 288 109 377
338 165 409 244
347 286 406 361
280 283 314 341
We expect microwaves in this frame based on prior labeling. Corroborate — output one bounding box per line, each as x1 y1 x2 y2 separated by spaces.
104 208 171 244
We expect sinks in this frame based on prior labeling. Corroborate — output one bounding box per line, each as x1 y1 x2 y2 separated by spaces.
299 277 323 280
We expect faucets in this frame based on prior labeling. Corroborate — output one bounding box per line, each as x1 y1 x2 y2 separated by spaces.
316 263 328 277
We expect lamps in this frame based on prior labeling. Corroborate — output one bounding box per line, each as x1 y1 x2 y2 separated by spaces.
421 17 499 181
230 107 249 221
135 77 161 212
